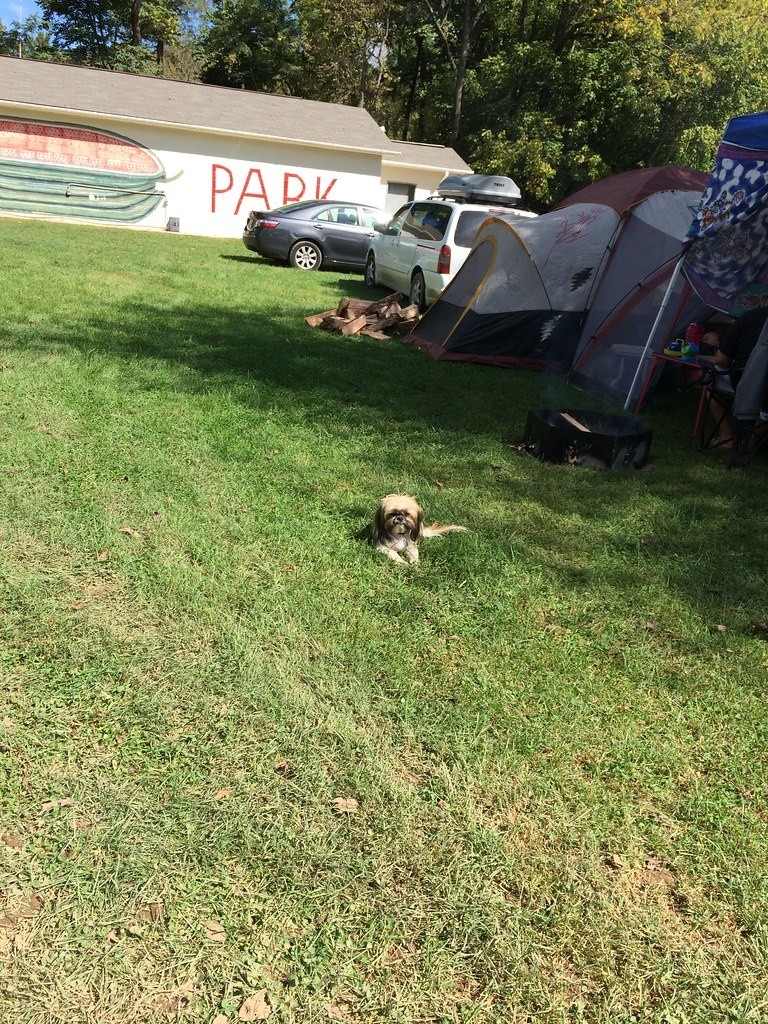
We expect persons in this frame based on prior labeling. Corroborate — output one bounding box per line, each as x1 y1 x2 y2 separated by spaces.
706 299 768 451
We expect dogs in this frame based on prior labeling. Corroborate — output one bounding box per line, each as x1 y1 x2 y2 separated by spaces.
363 492 471 566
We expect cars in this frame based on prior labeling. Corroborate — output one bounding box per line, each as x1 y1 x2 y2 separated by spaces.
242 199 401 273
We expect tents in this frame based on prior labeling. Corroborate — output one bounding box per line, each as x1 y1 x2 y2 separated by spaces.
399 165 757 417
622 108 768 415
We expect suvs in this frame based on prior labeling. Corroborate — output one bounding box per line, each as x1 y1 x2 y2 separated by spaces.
365 174 541 317
